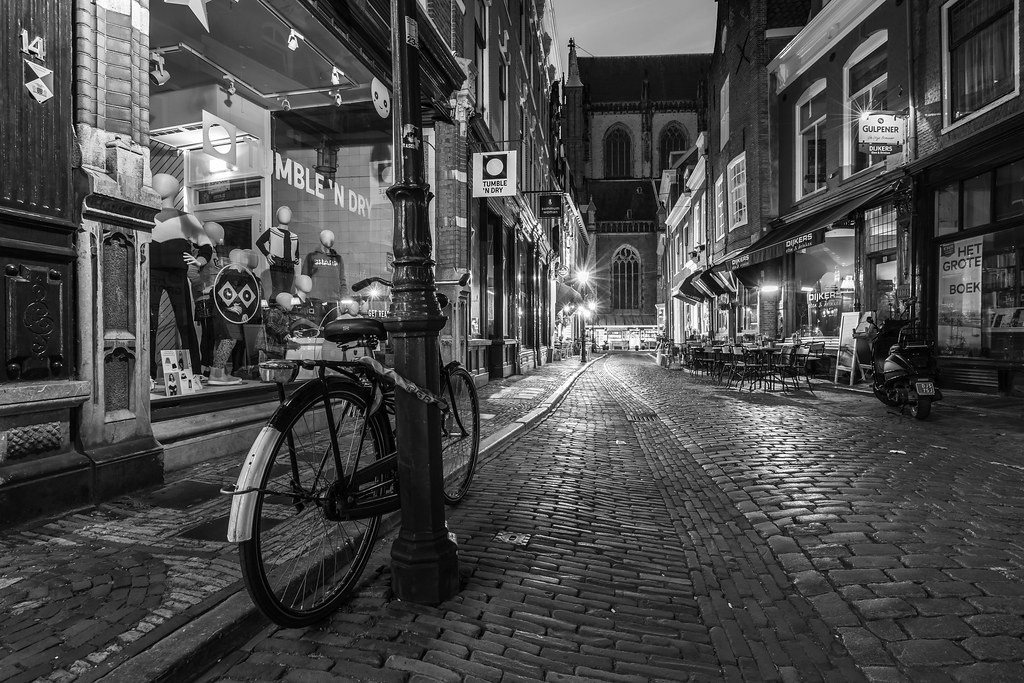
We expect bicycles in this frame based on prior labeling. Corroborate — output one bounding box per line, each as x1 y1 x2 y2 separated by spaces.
218 271 482 630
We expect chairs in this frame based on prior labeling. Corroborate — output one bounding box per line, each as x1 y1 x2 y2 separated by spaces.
666 340 827 395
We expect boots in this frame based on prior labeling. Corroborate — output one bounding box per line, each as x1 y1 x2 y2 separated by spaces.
208 366 242 385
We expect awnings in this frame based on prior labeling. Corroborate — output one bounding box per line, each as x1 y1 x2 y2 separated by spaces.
671 179 903 307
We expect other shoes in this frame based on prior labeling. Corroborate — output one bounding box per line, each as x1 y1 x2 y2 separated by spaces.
150 376 157 392
202 370 211 380
193 374 203 391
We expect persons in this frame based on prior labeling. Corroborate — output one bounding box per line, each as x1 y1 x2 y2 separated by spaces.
143 171 370 388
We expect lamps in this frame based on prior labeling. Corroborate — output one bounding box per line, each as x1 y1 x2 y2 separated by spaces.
281 99 290 111
150 53 170 85
331 67 340 85
332 94 342 107
222 75 236 95
287 29 299 51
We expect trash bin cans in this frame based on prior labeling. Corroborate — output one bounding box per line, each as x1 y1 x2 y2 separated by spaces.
657 349 664 366
661 354 668 366
671 347 679 359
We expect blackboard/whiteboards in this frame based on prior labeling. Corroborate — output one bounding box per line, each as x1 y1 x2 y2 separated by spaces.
835 312 866 386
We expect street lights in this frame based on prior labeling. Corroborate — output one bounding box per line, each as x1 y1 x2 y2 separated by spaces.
577 271 588 363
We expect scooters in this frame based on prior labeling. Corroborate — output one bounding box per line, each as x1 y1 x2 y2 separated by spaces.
865 298 935 419
